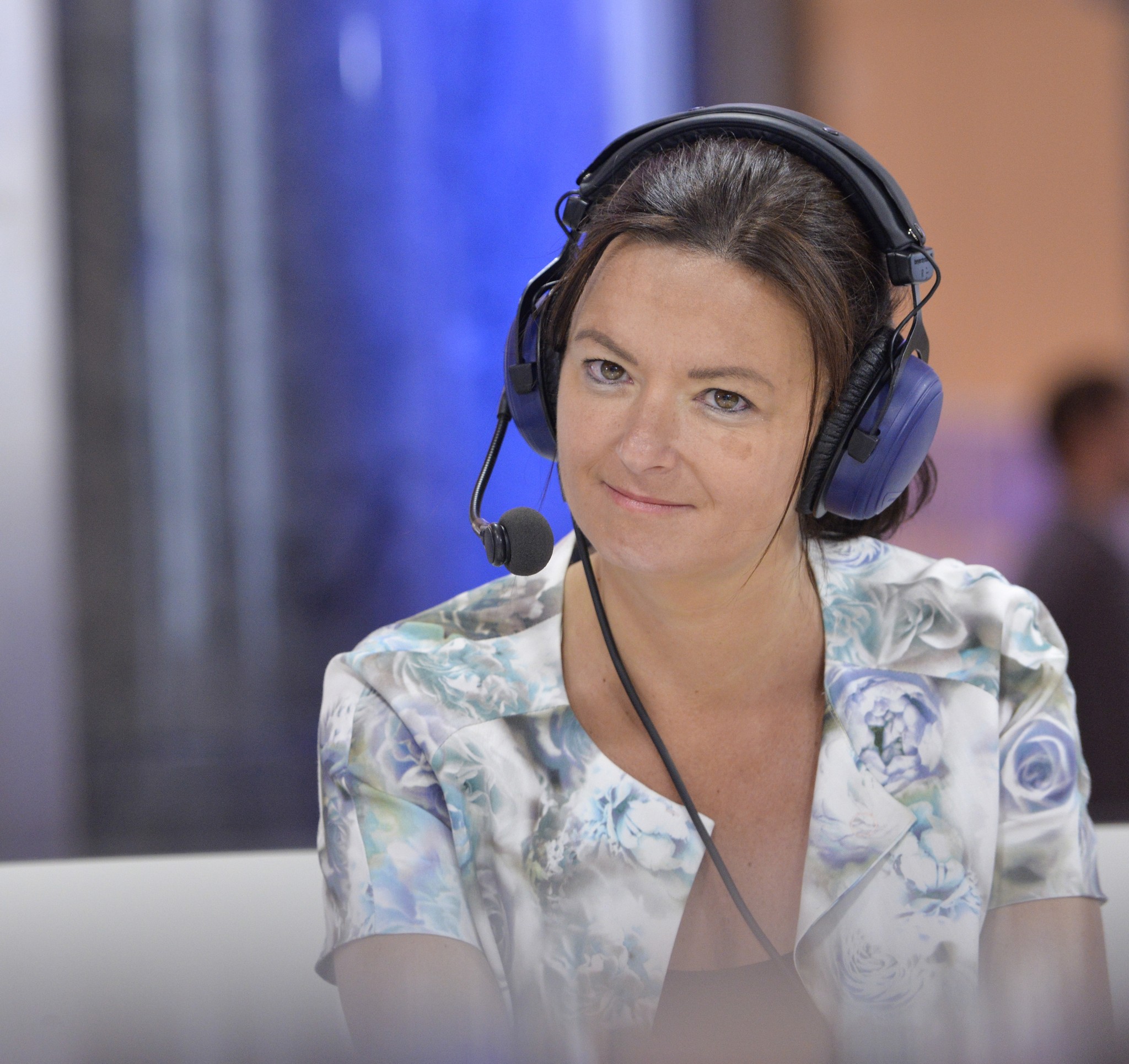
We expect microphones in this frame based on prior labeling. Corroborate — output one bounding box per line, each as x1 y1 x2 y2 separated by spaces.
470 390 554 576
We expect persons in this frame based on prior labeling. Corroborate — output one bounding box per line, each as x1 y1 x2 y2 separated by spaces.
316 103 1109 1064
1014 365 1129 825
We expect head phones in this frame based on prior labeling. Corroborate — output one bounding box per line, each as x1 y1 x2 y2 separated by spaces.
504 101 945 520
